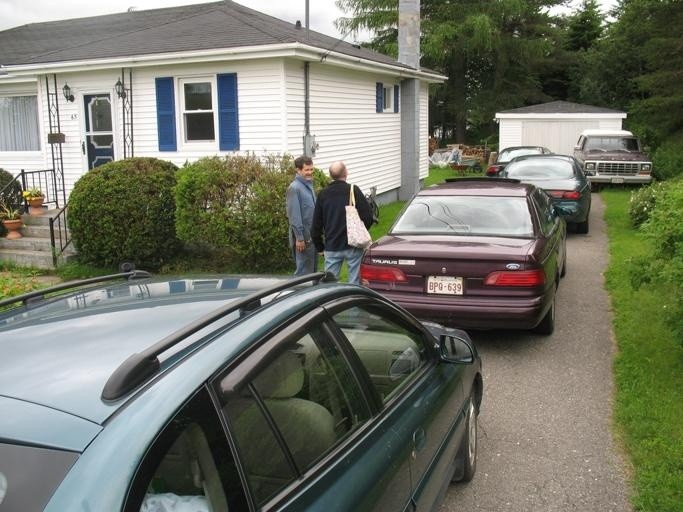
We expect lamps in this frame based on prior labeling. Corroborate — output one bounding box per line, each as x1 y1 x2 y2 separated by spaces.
62 83 75 102
115 79 126 98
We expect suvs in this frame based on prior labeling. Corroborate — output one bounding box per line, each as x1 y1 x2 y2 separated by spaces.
572 129 653 191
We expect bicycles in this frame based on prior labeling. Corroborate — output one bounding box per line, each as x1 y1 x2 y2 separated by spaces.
365 185 380 218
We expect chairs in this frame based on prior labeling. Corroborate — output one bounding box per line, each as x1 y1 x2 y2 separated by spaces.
149 422 230 512
221 349 338 502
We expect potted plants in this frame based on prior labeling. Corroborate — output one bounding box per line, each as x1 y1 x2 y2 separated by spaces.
0 206 23 240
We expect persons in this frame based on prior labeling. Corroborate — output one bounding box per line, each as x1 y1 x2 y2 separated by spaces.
283 155 319 275
310 161 376 286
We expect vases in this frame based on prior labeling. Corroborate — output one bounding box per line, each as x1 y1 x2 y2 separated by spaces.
28 197 45 215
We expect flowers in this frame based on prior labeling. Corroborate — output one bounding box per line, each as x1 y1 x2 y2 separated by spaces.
22 186 43 200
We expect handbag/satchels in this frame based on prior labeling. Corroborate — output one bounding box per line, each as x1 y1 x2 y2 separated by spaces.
345 184 372 248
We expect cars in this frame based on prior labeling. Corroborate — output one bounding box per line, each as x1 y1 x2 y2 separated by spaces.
359 174 568 337
498 154 596 234
485 146 551 178
2 269 485 512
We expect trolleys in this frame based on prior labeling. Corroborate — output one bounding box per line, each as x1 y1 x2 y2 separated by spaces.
461 157 483 174
